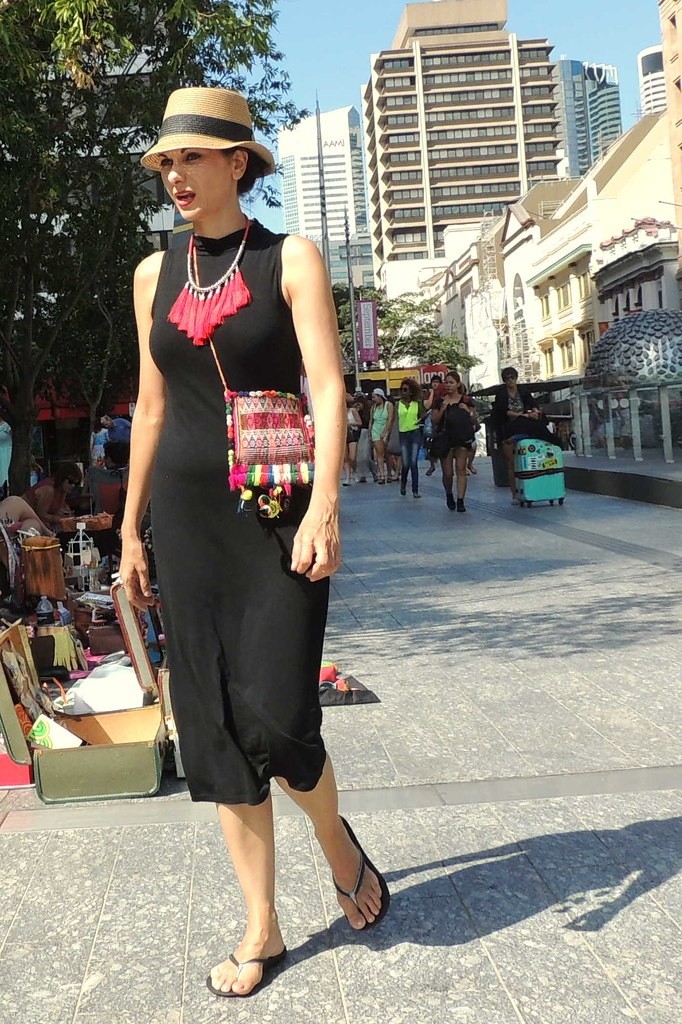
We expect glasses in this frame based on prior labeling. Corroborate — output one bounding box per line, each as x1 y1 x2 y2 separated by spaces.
400 388 410 391
506 375 516 381
66 477 79 487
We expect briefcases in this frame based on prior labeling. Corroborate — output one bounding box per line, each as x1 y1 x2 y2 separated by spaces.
0 618 166 804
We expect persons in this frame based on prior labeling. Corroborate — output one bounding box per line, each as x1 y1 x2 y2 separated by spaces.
492 367 549 505
0 415 156 601
342 371 477 512
119 87 390 997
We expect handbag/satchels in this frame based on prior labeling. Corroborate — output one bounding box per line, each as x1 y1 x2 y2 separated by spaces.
424 414 434 437
225 389 313 488
387 401 401 454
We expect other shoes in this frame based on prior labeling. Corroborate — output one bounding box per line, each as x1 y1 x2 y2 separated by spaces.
413 491 421 498
511 497 519 506
467 464 476 474
446 493 457 511
457 498 466 512
426 467 435 476
342 472 400 486
465 467 470 476
401 483 406 494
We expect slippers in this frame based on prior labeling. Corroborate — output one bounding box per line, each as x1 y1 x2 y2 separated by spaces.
327 815 390 931
207 944 286 995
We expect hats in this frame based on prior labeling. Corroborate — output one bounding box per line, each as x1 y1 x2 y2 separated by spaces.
371 388 387 400
141 87 277 179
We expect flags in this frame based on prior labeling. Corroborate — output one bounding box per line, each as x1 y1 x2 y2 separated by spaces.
356 300 378 361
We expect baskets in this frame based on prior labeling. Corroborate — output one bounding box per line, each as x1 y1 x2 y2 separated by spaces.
59 514 114 532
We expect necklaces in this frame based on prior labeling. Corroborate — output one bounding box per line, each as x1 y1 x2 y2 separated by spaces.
165 212 253 347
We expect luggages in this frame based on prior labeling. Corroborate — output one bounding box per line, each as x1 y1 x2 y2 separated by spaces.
516 411 566 508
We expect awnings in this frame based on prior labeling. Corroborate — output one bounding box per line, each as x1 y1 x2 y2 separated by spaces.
36 402 136 421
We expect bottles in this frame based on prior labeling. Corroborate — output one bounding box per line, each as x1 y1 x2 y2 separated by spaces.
37 596 55 628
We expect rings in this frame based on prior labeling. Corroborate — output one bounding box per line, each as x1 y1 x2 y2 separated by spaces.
322 569 325 573
